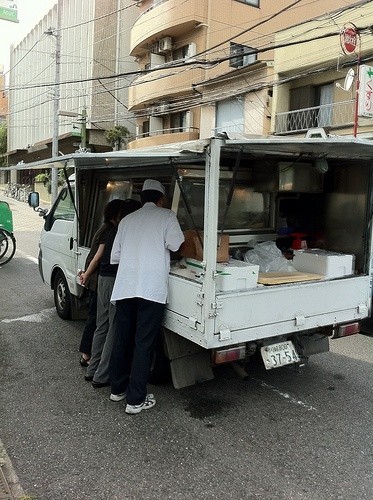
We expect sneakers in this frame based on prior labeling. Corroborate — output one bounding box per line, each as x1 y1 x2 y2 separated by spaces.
125 394 156 414
109 392 126 402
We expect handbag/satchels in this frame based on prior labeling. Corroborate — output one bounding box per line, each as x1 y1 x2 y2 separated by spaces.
244 240 297 272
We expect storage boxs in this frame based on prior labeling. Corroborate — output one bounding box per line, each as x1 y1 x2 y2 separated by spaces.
187 258 260 292
293 248 353 278
182 232 229 262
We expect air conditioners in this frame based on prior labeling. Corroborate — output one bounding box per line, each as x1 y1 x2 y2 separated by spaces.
158 36 172 53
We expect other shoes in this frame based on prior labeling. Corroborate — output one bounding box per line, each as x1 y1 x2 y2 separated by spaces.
80 356 91 366
84 375 94 381
92 381 111 388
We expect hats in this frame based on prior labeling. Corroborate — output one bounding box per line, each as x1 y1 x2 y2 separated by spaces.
142 179 167 197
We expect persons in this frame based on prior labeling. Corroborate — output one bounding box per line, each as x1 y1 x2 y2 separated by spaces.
109 180 185 415
79 200 142 388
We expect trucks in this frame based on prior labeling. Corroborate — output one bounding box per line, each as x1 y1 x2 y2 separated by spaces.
0 128 373 390
